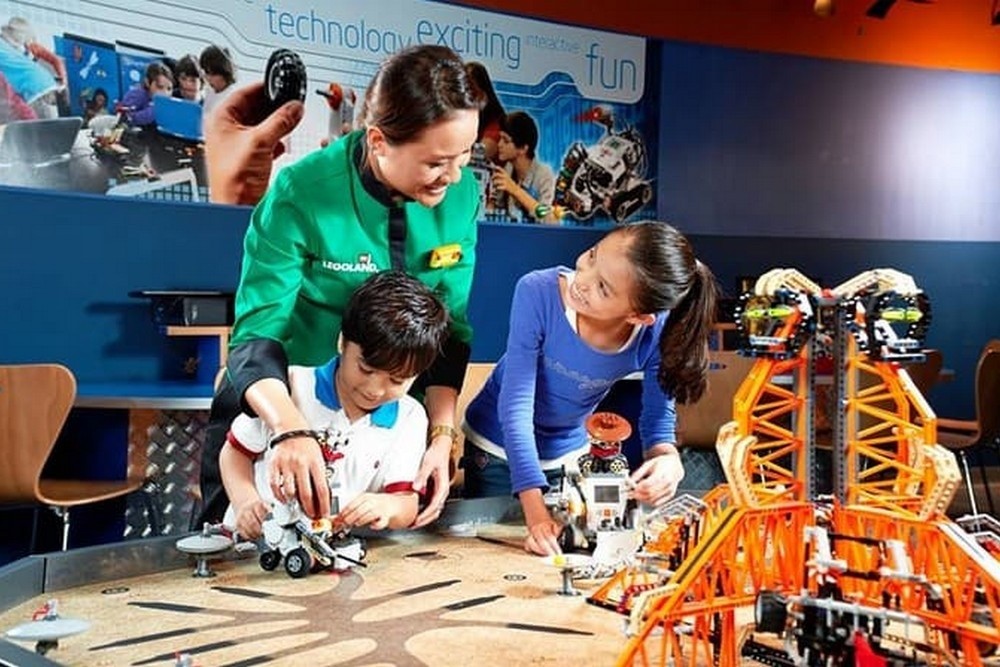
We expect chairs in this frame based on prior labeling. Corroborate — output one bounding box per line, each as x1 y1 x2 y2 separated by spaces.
0 363 143 553
670 349 1000 533
421 362 499 486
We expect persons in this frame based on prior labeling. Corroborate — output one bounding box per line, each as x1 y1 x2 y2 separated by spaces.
194 44 485 534
0 17 303 204
468 57 556 218
460 222 716 556
219 270 447 541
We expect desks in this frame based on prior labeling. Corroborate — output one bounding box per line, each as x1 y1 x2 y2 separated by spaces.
0 489 726 667
72 385 215 542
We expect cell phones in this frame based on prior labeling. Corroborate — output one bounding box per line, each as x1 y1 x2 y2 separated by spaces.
129 289 222 296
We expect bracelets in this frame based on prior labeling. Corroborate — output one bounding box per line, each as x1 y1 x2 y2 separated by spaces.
270 429 319 451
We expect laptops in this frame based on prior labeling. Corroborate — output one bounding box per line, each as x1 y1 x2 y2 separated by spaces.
153 94 202 141
0 118 83 165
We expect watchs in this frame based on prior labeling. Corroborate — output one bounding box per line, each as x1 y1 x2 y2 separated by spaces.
431 425 459 457
